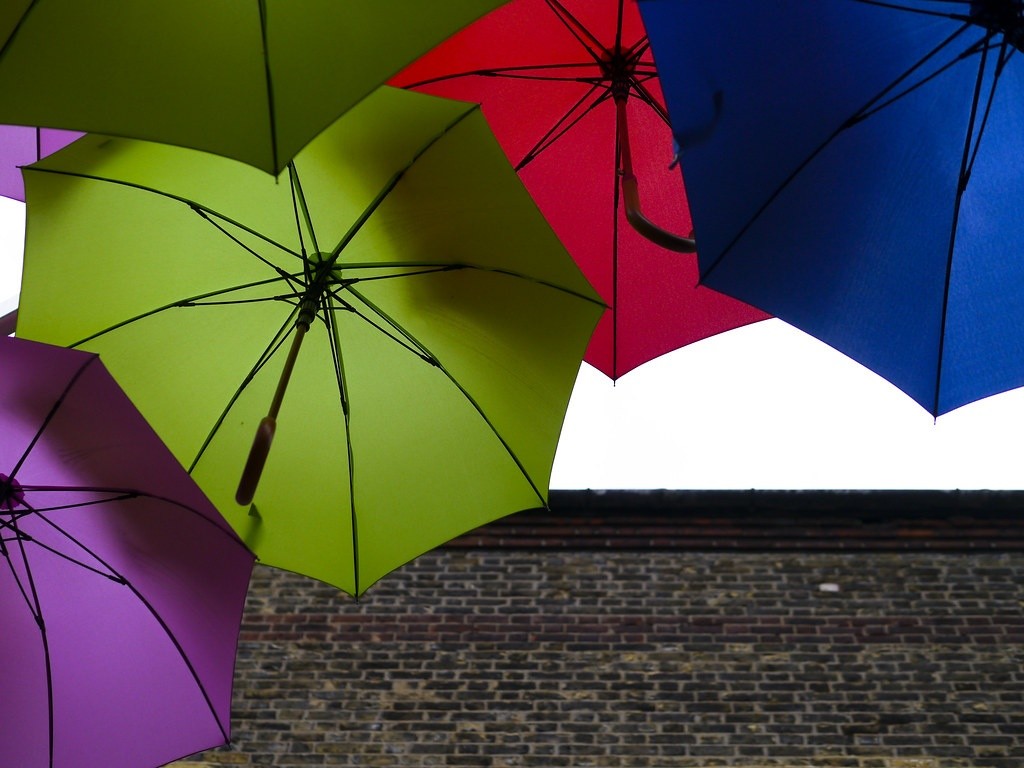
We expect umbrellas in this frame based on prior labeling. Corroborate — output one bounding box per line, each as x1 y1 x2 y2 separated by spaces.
0 0 1023 768
